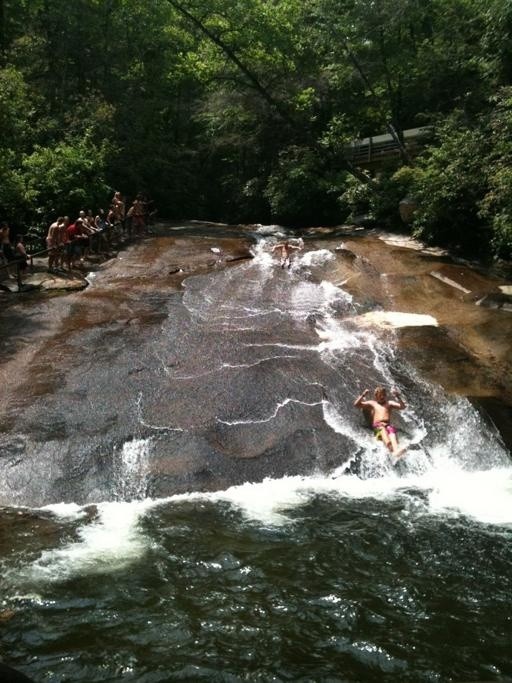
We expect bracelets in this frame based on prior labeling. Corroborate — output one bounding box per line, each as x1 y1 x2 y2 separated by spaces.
360 393 365 397
396 398 401 402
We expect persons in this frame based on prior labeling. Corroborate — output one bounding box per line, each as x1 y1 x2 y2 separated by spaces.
45 192 147 268
273 241 300 269
354 387 406 456
0 222 28 286
